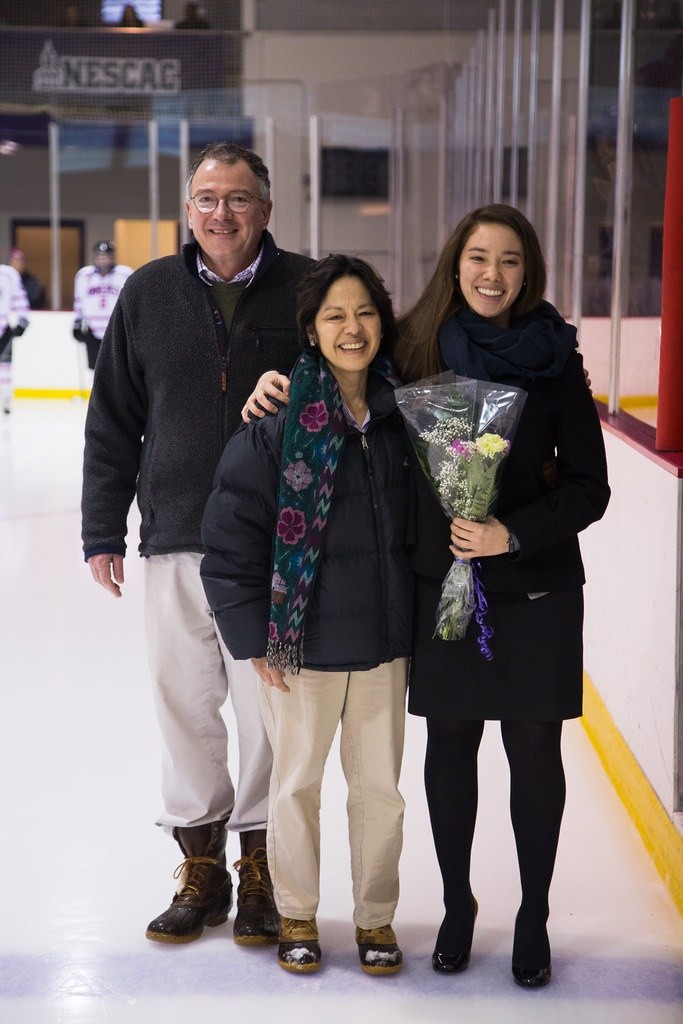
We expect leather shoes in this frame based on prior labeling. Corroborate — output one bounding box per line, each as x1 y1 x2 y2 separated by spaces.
512 902 551 986
432 894 478 974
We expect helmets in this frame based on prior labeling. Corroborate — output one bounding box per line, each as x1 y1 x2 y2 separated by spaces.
92 239 116 276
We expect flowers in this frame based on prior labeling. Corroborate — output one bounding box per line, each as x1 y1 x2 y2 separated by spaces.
417 415 511 641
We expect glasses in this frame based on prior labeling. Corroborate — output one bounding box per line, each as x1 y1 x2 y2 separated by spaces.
191 188 265 215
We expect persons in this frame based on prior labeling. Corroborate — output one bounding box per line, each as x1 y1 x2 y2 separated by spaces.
10 250 48 310
241 202 611 987
81 141 318 946
0 265 29 414
198 254 559 975
75 240 135 373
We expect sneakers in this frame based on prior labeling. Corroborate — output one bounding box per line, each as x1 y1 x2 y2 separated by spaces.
277 913 323 973
355 921 404 976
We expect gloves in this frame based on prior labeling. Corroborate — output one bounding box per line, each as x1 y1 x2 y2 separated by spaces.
11 325 26 337
73 320 86 341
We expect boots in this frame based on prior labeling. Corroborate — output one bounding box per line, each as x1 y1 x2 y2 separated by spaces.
146 819 232 941
234 829 279 948
